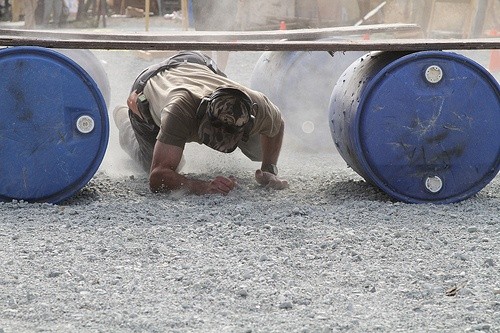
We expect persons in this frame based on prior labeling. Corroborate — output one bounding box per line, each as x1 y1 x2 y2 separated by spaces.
113 50 290 194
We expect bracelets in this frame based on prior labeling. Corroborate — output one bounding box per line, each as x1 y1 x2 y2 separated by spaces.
262 162 279 175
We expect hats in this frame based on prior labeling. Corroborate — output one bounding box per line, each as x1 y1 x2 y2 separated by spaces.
198 85 254 153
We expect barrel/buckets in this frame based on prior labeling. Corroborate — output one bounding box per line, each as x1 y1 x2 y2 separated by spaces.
36 32 112 114
328 48 500 204
249 36 360 152
0 41 113 205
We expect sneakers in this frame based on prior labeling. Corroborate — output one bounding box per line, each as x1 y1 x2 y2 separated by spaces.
114 105 134 156
